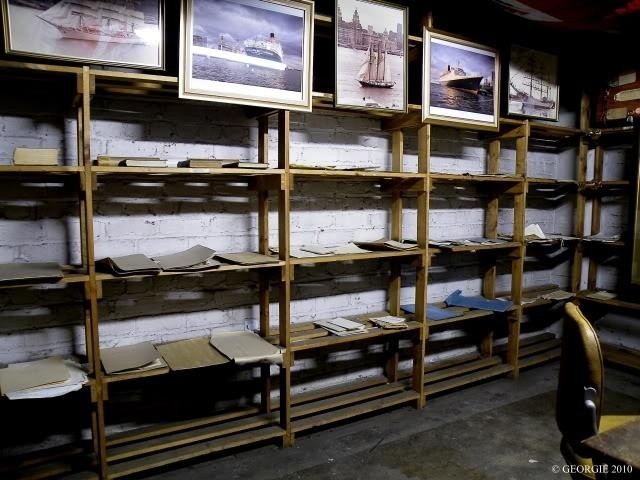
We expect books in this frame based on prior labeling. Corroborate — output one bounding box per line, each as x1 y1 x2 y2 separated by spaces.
97 157 160 166
177 159 223 167
222 162 269 169
13 147 58 166
118 159 169 168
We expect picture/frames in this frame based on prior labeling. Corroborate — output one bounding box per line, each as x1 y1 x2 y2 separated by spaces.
2 2 166 71
507 63 560 121
334 1 408 113
178 0 314 112
422 27 500 133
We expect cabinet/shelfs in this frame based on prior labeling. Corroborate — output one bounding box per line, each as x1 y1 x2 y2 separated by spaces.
1 62 640 479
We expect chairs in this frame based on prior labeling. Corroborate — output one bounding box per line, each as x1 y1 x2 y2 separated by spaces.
556 301 639 479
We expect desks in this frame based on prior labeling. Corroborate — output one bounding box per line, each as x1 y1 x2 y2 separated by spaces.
581 419 639 478
